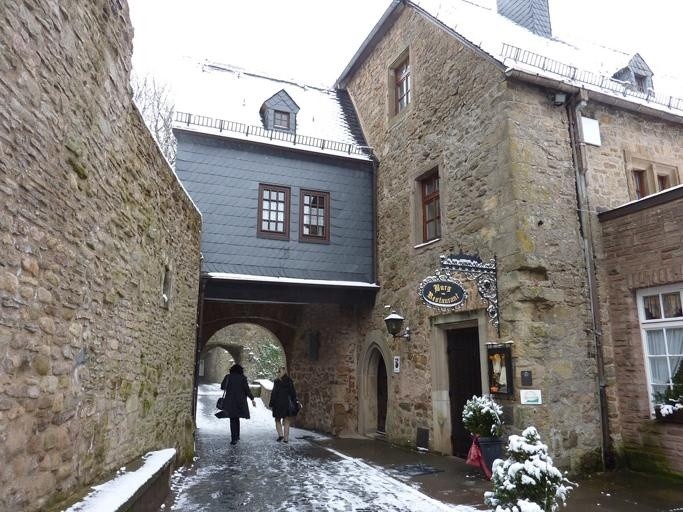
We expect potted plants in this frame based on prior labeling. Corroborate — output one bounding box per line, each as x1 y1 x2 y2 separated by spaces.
462 392 506 472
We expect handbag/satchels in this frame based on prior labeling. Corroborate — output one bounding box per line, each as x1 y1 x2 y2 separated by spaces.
290 400 300 412
216 398 225 410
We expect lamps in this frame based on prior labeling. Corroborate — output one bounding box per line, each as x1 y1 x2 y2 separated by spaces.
382 309 410 343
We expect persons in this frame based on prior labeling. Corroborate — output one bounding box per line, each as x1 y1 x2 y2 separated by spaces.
222 365 256 446
268 367 302 443
